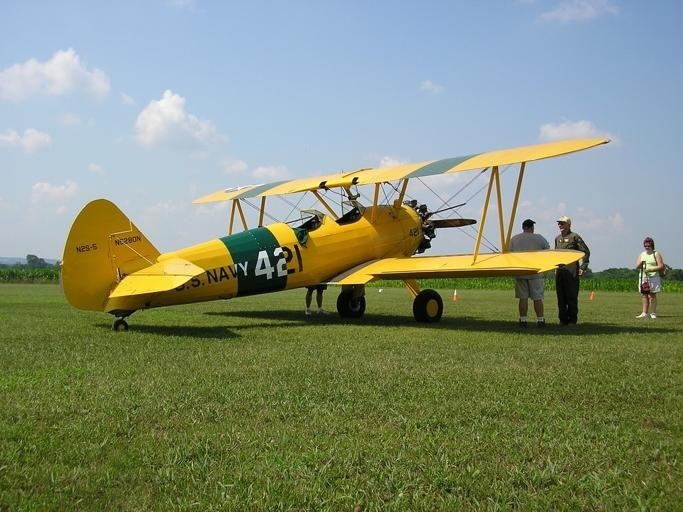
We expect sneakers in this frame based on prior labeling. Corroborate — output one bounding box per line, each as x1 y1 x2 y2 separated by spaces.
304 308 330 316
635 312 656 319
517 316 577 328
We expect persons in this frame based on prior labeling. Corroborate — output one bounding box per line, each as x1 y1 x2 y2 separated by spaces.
304 284 329 316
553 216 589 328
509 219 549 327
636 237 672 318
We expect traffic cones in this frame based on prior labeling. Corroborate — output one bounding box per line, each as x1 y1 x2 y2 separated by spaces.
590 290 596 301
452 288 460 302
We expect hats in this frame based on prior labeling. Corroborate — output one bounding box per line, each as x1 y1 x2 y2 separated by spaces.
522 219 536 228
555 216 571 226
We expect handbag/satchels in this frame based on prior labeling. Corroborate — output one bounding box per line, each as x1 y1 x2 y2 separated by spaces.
653 251 666 278
640 282 650 294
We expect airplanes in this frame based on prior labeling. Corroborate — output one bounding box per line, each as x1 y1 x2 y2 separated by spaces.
62 138 612 333
